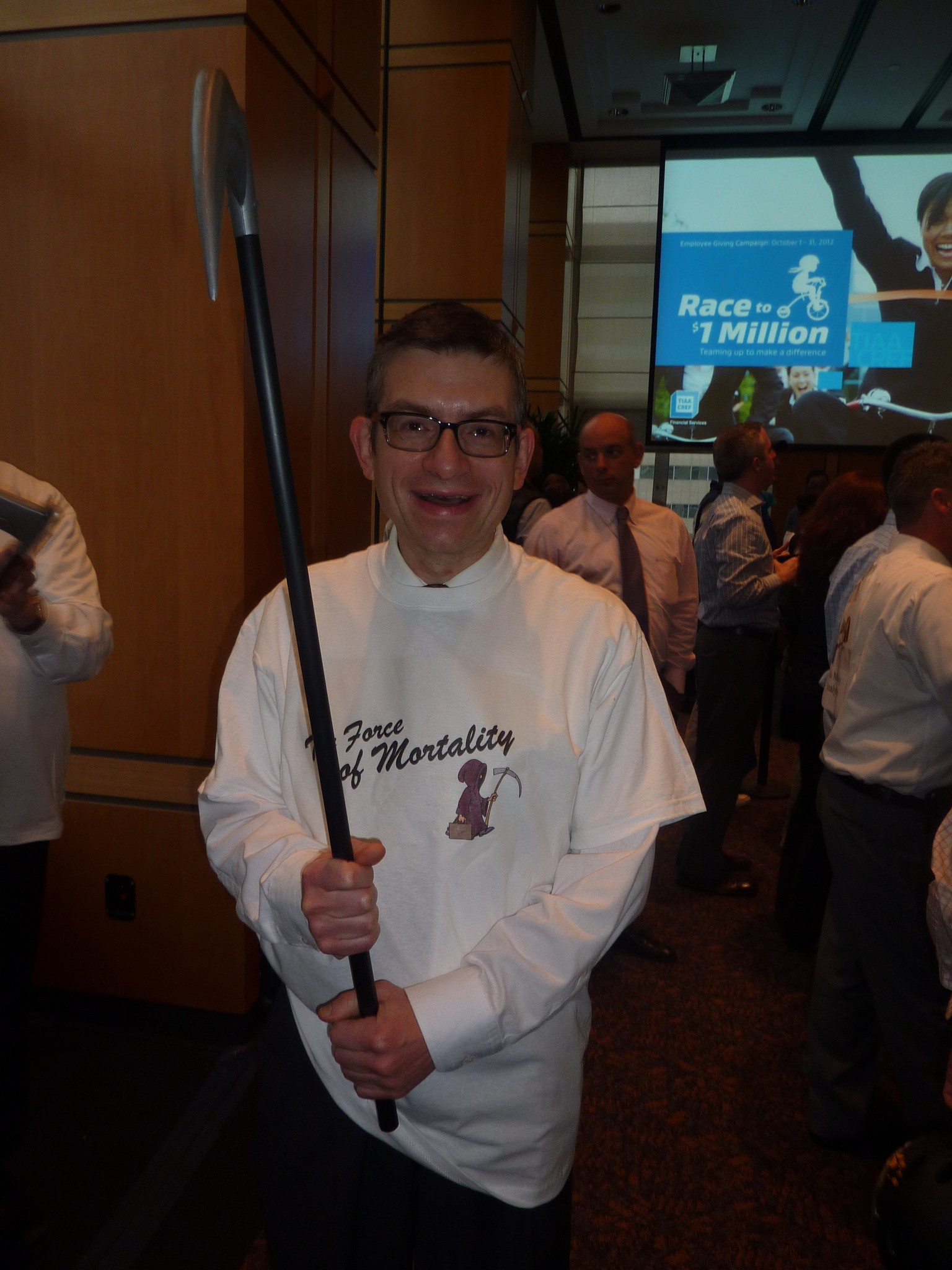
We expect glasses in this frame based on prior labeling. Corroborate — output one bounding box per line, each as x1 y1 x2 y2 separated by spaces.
370 410 521 458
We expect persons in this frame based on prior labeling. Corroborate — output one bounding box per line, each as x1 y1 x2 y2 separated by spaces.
197 301 705 1270
776 433 950 960
516 474 569 546
678 421 800 891
653 366 814 427
523 412 700 712
814 154 952 433
0 460 115 1020
808 441 952 1162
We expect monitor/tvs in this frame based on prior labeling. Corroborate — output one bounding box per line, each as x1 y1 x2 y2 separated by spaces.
642 124 952 451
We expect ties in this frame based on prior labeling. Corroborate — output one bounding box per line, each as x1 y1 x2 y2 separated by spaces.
617 506 650 650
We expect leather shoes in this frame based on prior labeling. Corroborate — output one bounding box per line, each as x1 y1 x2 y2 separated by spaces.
723 851 751 870
714 882 752 895
618 931 674 961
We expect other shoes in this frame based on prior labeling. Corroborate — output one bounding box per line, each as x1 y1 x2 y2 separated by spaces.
735 794 751 808
